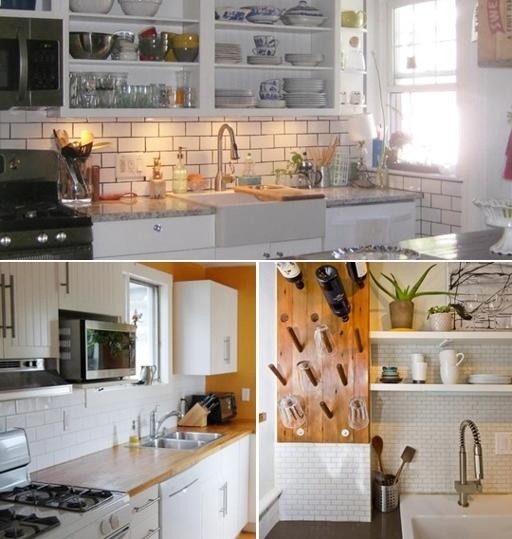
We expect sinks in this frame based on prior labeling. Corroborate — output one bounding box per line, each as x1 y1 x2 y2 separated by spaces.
168 190 327 248
399 494 512 539
162 431 224 442
136 438 206 451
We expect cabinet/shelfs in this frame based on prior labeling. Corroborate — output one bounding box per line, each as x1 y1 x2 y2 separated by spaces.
172 280 238 376
91 210 215 260
0 263 60 359
58 262 123 316
130 436 249 539
0 0 365 118
368 331 512 393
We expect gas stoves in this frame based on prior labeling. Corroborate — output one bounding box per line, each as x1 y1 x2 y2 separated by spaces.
0 194 92 246
0 481 131 539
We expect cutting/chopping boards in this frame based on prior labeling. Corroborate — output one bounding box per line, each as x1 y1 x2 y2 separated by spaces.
234 184 325 201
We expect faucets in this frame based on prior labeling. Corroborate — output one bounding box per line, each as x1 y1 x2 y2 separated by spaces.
460 419 484 505
150 411 184 437
215 123 240 191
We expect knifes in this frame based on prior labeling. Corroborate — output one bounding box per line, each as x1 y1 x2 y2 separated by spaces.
201 394 219 410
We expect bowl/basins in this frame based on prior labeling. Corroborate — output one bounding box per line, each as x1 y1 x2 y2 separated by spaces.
216 1 281 26
68 0 199 61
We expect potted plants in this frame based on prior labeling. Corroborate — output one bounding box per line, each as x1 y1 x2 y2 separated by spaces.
427 305 455 332
370 264 457 329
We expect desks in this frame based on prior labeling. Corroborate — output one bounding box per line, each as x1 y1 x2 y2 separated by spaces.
264 227 512 260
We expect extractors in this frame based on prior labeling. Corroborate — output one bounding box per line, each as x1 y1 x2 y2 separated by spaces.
0 359 74 402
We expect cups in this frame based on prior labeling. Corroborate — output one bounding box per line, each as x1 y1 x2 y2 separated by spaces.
252 35 284 100
69 70 198 108
279 394 306 430
438 350 465 384
342 10 368 28
60 156 93 199
313 324 339 359
297 360 320 393
347 395 371 431
409 354 429 383
316 167 330 188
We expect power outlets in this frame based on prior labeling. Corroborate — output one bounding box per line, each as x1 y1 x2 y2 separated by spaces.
241 388 250 401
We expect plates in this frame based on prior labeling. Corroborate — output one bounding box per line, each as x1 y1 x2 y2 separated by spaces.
379 366 404 383
327 243 422 260
216 43 328 108
468 374 512 384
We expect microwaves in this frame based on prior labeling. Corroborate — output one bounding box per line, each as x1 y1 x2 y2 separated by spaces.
192 391 238 424
60 319 137 380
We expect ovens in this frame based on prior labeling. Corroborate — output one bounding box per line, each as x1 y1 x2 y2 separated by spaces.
1 17 64 108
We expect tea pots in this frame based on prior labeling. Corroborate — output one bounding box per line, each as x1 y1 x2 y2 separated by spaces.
140 364 157 385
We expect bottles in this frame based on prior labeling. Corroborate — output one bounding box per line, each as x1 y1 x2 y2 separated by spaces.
314 264 352 323
242 153 256 177
350 90 365 104
277 262 304 290
345 262 369 290
372 131 383 167
339 91 347 104
149 157 167 199
